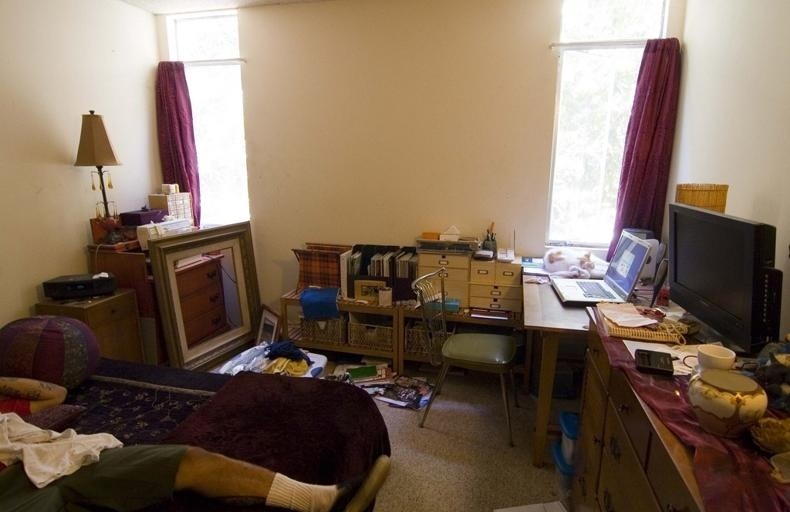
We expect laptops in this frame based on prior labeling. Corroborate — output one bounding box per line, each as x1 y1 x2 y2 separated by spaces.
552 230 652 307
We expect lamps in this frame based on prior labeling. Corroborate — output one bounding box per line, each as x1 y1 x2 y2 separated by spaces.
75 109 130 249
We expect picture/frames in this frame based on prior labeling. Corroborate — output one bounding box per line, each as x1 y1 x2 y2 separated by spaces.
146 220 281 371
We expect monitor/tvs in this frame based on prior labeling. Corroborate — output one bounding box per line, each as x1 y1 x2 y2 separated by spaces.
668 202 783 356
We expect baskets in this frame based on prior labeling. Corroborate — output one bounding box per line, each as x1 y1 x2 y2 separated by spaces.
298 315 347 345
347 321 394 351
405 319 457 354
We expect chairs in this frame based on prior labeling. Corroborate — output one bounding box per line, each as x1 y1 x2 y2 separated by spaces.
409 264 526 449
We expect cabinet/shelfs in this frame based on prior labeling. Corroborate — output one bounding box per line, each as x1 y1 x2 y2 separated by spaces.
280 242 521 378
34 242 229 365
575 304 790 512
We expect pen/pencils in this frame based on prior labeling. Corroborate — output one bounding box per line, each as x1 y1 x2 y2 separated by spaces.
487 229 497 241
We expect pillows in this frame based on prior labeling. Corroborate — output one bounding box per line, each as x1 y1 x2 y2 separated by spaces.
20 403 87 432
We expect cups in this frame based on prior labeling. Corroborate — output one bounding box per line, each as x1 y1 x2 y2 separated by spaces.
697 344 736 370
483 240 497 259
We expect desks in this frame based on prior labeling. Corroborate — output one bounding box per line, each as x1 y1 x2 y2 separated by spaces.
521 263 661 467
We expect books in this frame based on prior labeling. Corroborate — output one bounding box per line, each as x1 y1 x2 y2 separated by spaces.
330 246 440 412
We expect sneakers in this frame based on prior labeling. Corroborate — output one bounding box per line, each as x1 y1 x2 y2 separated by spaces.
328 454 390 511
